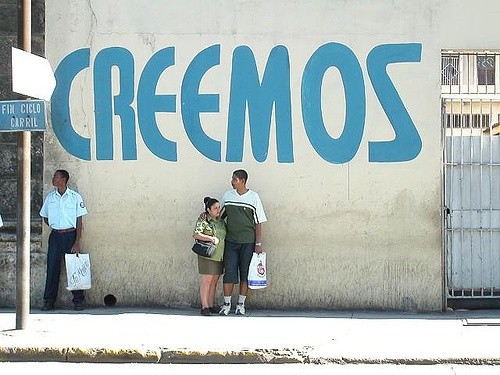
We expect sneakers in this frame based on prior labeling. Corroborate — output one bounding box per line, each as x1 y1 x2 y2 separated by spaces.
218 302 231 315
74 303 82 310
209 306 218 313
234 303 245 315
201 305 211 315
41 302 56 310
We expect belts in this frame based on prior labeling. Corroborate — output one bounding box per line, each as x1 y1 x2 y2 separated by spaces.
53 229 76 234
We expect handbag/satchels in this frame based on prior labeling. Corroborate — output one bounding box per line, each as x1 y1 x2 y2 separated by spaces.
248 251 268 289
192 219 218 257
65 252 91 291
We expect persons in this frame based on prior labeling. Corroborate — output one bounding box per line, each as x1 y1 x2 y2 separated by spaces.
193 197 227 315
200 170 267 316
40 170 88 310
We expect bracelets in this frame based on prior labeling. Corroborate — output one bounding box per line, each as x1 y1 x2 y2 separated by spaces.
255 243 262 246
